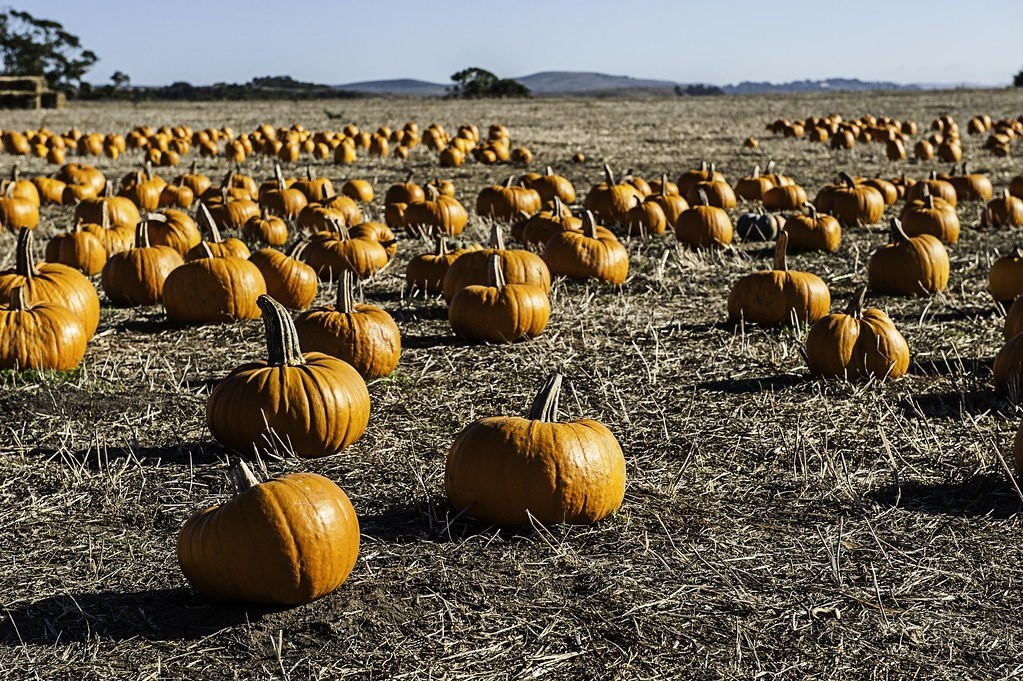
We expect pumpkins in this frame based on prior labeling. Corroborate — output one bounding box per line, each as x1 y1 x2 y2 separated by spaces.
0 113 1023 610
